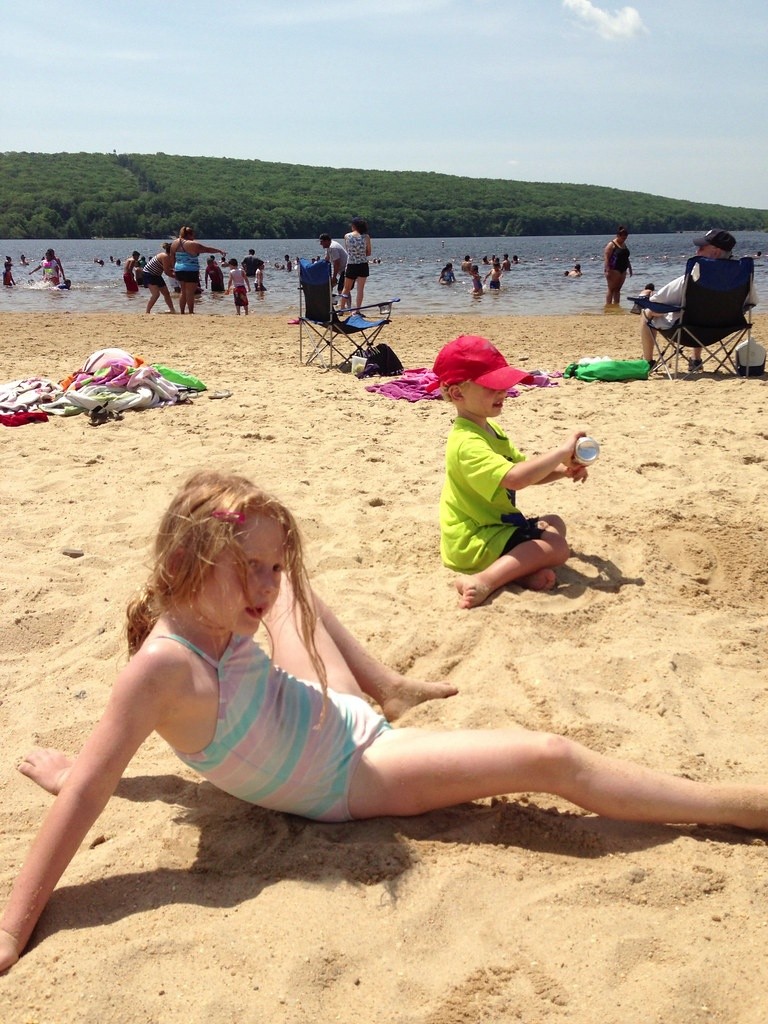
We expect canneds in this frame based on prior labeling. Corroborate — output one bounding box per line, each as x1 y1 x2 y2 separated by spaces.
571 436 600 466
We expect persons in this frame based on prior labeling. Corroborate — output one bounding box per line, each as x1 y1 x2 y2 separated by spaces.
339 217 371 317
638 283 654 296
0 472 768 972
564 263 582 277
93 251 153 293
439 253 518 292
371 258 382 264
141 243 176 314
3 248 67 287
170 227 228 314
169 249 320 293
225 258 251 315
320 233 355 315
432 334 590 610
603 226 633 304
641 228 760 375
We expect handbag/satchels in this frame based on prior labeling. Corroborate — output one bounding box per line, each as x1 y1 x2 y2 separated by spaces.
563 359 650 382
363 343 404 377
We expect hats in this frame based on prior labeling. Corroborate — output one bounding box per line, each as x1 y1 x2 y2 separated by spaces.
432 335 531 391
692 229 736 252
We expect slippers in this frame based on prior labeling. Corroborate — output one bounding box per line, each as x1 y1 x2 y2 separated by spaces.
183 387 204 398
208 388 233 399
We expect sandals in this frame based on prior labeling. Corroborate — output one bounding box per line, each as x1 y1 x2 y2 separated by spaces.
88 402 122 426
173 394 194 405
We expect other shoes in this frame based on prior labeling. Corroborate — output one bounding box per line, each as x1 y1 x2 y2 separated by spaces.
688 357 703 372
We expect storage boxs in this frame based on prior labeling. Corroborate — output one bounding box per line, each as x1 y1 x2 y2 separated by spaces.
736 337 765 377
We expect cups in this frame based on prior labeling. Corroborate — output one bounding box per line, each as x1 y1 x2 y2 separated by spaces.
351 355 367 376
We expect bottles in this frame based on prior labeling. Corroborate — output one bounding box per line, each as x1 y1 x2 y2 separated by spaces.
573 437 600 467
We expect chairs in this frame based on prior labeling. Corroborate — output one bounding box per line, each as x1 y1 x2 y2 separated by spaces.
627 256 755 383
297 258 401 371
